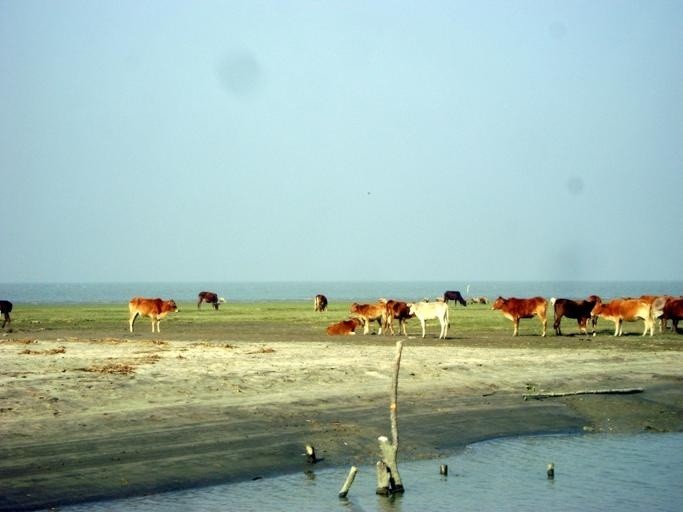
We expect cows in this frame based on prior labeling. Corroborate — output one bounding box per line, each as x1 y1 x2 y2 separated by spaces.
491 296 549 337
197 291 219 310
552 295 683 337
129 296 180 333
407 301 451 340
325 297 416 340
470 296 488 305
443 291 467 306
315 294 328 311
0 300 12 328
